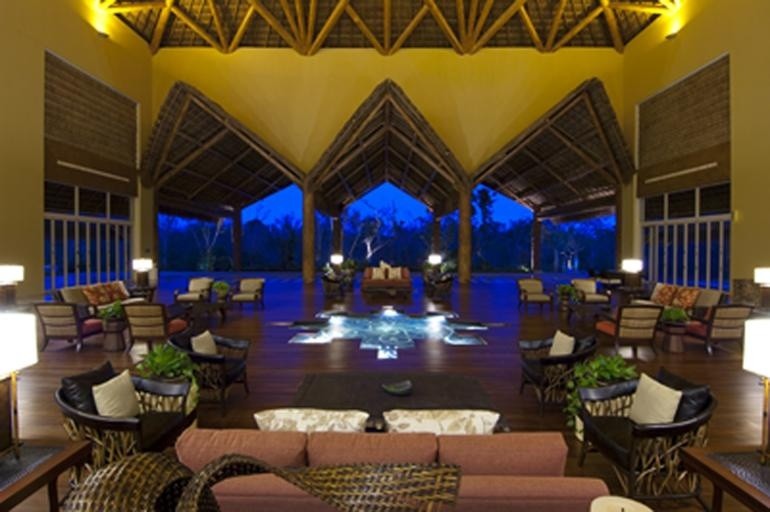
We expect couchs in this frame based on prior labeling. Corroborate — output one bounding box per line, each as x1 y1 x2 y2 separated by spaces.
361 267 411 291
177 428 610 512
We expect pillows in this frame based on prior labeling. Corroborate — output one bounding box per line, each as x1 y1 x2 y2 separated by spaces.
371 267 403 280
254 409 501 435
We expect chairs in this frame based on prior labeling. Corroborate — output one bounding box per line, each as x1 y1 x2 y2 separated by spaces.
322 250 354 298
423 254 454 302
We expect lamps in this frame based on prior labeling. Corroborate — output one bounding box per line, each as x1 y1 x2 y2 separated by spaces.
622 260 644 289
754 267 770 307
0 265 25 307
133 259 152 287
742 318 770 465
0 310 39 458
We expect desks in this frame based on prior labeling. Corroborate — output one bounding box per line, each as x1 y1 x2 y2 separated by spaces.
0 439 92 512
678 445 770 512
365 284 407 302
